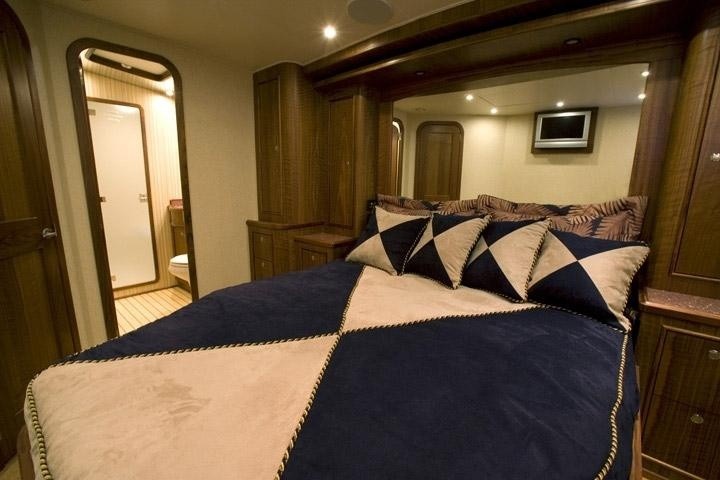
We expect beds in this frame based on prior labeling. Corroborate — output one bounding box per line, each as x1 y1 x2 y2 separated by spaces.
17 258 642 479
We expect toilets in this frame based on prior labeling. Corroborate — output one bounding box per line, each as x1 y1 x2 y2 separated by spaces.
168 254 190 287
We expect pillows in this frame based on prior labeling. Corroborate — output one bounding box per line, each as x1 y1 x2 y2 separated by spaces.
403 212 490 290
345 205 430 277
529 229 650 334
461 217 552 303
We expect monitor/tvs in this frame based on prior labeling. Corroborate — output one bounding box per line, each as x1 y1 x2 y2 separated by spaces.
534 110 592 151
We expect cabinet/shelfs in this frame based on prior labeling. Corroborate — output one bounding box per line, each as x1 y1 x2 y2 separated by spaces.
170 211 191 294
633 1 719 480
243 63 382 286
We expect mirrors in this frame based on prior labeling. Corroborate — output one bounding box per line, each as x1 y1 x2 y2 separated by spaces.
379 62 651 217
